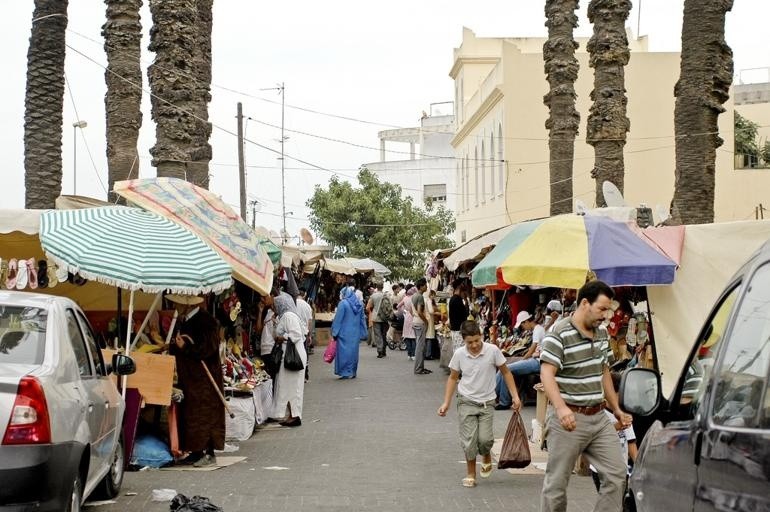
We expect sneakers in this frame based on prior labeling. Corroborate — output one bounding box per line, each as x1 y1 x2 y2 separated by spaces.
279 416 301 427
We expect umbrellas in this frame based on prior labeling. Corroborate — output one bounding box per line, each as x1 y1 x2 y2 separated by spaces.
111 177 275 297
469 211 680 304
40 204 234 400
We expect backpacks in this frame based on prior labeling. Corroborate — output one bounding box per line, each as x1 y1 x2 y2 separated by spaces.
377 291 394 322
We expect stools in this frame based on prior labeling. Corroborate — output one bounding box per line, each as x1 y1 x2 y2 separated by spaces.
517 369 540 404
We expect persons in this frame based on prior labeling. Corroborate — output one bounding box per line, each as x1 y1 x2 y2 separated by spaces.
332 286 368 379
449 280 469 379
495 299 565 411
438 321 521 486
165 293 225 467
333 282 366 304
540 281 638 512
366 277 438 374
257 287 313 427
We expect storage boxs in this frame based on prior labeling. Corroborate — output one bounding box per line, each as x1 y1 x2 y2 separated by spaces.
311 325 334 345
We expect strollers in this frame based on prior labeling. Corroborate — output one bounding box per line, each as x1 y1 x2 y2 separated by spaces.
386 313 408 351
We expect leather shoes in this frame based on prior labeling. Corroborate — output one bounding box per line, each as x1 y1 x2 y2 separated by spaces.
176 453 217 467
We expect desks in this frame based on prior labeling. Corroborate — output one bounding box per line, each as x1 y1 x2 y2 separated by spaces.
225 374 273 442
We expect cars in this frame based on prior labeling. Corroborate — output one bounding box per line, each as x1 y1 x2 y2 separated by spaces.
0 288 137 512
620 237 770 512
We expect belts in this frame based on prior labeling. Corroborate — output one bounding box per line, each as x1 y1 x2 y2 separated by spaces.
548 399 607 416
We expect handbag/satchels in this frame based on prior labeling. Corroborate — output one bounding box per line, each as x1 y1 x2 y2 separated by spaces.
267 339 283 373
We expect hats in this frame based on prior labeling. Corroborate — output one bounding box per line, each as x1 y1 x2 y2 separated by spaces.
164 294 205 305
513 311 533 331
547 299 562 311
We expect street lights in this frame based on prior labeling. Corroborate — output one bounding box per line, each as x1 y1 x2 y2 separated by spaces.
72 119 88 196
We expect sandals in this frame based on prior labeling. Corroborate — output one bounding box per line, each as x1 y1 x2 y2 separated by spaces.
419 368 433 374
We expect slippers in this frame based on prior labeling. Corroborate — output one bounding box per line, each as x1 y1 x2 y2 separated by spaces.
1 257 88 289
462 475 478 487
480 461 493 478
